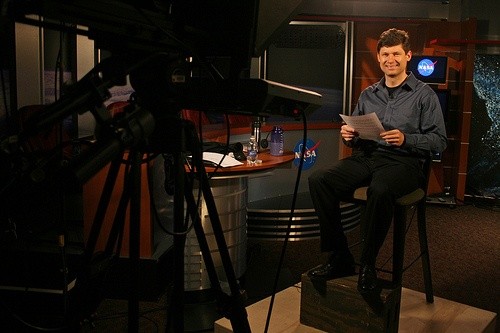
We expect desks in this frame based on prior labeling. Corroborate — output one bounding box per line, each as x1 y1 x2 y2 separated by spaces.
185 148 295 175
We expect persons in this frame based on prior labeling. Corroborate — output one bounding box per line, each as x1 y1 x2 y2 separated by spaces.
307 29 448 300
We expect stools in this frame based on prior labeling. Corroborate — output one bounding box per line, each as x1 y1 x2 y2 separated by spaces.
340 154 433 333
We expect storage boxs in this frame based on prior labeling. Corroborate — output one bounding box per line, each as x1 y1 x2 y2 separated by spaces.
299 270 393 333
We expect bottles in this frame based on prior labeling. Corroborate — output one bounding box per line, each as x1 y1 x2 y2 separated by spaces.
269 125 284 156
251 116 261 143
248 136 258 163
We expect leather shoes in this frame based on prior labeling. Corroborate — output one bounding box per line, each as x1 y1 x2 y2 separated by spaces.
307 254 355 281
357 272 380 296
